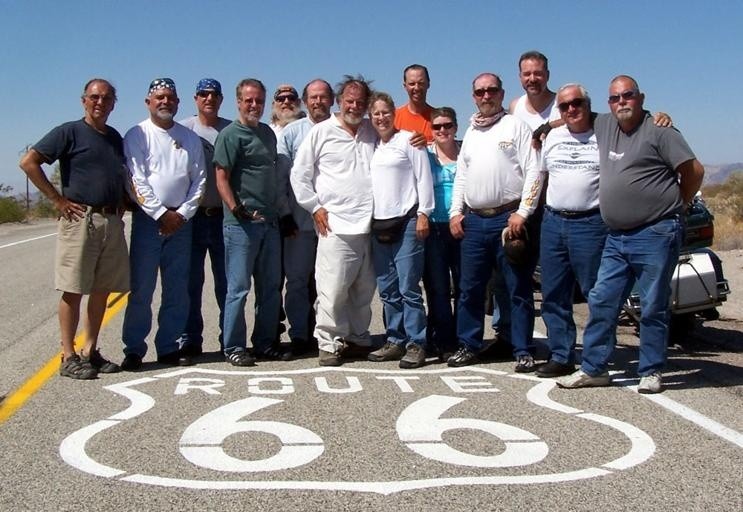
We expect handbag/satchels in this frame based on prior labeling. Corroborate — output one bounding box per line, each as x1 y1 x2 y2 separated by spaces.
371 216 409 245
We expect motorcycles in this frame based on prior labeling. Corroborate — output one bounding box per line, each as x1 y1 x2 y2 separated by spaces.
617 183 731 353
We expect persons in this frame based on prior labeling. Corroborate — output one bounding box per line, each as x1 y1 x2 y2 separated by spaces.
274 79 334 355
178 78 235 360
535 83 672 377
425 107 462 360
269 84 318 351
367 93 436 370
122 78 208 372
289 75 428 366
383 65 436 349
480 51 561 363
19 79 131 380
212 79 294 366
531 75 705 395
447 73 545 373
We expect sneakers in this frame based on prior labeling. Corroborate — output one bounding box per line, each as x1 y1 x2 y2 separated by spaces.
120 354 142 371
252 337 316 361
318 349 343 366
58 355 97 379
514 354 535 372
347 342 383 359
399 343 426 369
533 359 575 377
366 343 403 362
220 345 256 367
156 345 202 366
79 349 119 373
437 337 512 365
555 368 611 388
637 376 661 394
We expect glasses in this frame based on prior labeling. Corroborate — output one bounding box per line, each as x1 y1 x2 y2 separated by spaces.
557 98 586 112
275 95 297 102
609 91 638 104
149 79 174 89
196 90 222 98
473 87 500 97
431 122 455 131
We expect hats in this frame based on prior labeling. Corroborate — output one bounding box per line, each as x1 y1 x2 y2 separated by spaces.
500 225 529 266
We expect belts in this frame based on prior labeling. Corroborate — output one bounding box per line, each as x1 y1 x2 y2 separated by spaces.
544 205 599 219
81 206 119 215
468 200 519 218
198 207 219 217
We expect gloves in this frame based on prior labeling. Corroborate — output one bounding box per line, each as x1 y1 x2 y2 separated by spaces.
277 213 299 233
232 204 252 226
531 121 552 144
